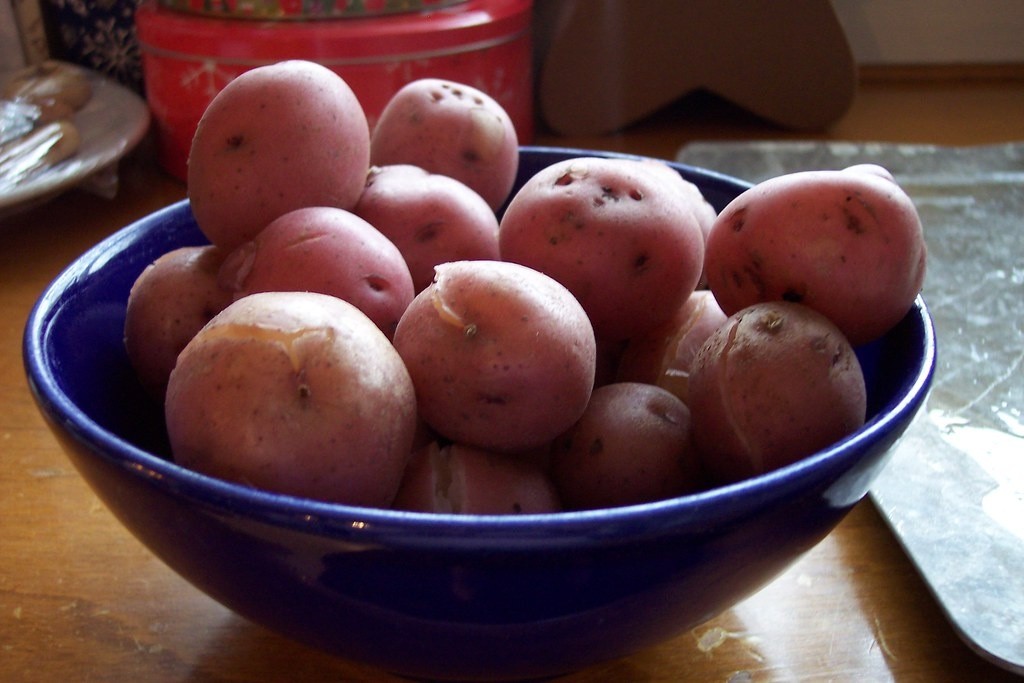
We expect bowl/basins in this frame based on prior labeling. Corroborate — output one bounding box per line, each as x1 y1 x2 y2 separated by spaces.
21 147 937 683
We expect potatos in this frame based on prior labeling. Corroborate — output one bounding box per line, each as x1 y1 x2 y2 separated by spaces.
125 61 927 514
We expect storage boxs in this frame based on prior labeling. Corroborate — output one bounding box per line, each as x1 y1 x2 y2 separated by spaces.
132 0 538 193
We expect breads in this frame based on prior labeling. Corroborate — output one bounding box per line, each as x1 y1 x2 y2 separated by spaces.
0 56 91 189
